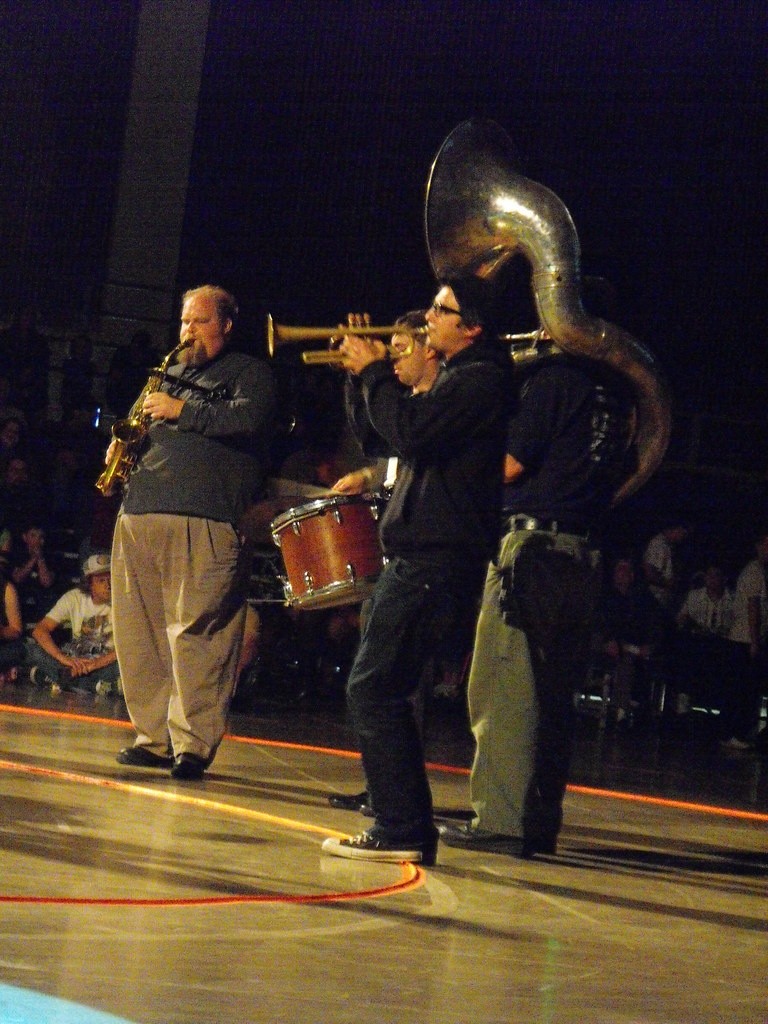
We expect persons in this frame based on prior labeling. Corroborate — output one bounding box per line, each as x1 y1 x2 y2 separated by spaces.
103 284 279 782
436 276 630 855
325 306 445 818
316 270 513 868
0 304 768 763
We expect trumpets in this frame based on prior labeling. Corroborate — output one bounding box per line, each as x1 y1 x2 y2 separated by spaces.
265 314 430 360
302 313 397 363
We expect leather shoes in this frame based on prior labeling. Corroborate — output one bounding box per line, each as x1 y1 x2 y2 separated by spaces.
115 745 175 766
173 755 205 780
328 788 375 818
435 823 528 857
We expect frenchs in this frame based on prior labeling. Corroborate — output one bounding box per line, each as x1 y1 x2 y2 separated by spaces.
424 110 673 509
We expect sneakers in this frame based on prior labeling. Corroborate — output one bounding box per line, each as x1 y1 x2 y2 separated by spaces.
321 837 423 865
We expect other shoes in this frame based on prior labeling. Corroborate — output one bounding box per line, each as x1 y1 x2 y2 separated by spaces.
723 736 749 749
1 666 19 682
95 678 112 695
31 666 57 690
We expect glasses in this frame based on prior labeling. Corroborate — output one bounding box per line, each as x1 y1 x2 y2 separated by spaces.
431 300 464 317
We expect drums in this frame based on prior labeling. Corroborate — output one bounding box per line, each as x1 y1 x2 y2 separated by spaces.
270 491 384 611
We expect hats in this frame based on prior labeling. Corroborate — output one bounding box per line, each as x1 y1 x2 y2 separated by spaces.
84 553 113 576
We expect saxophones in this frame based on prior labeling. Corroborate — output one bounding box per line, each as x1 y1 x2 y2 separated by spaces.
96 338 195 496
